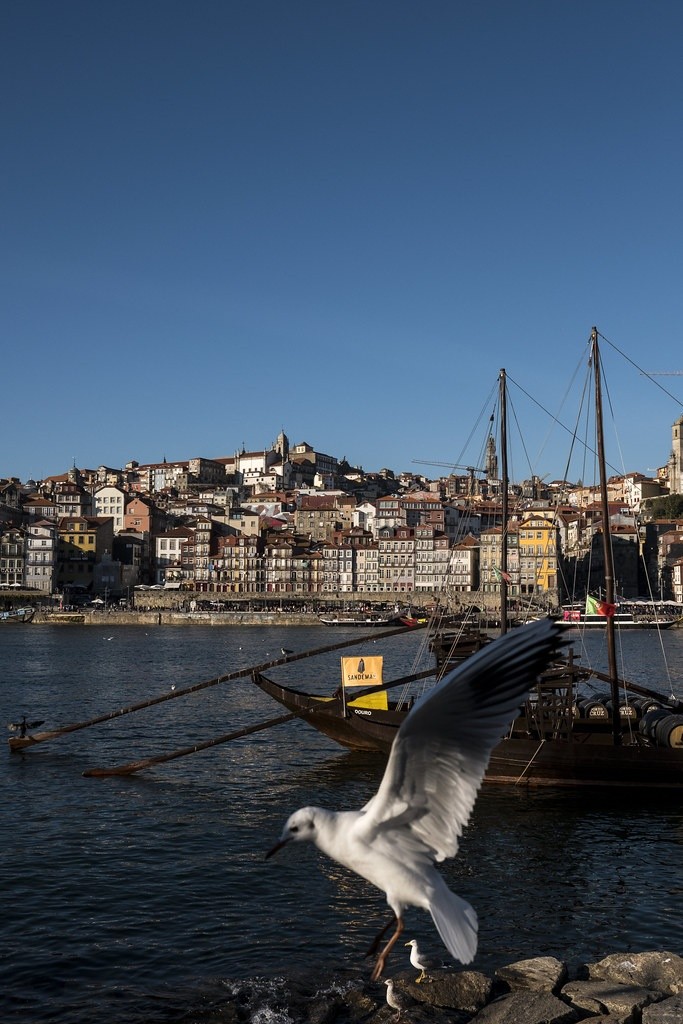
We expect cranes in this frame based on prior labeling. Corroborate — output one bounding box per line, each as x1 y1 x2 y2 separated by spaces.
410 457 487 496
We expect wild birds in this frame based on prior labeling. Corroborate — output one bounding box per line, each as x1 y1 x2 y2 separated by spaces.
265 613 576 981
384 979 427 1023
102 633 270 690
405 939 453 984
281 647 294 657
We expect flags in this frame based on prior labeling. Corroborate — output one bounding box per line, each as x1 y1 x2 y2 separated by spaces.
343 657 384 687
585 594 616 617
491 564 513 582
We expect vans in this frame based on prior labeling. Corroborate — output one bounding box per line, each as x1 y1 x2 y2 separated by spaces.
120 598 126 606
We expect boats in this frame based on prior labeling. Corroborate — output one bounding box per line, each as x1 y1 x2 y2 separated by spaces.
531 605 682 629
448 618 523 629
400 617 454 628
319 615 388 625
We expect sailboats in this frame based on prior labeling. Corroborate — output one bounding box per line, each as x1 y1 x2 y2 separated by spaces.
249 324 683 794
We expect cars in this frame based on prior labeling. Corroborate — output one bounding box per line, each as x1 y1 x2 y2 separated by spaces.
151 586 168 591
136 585 150 591
